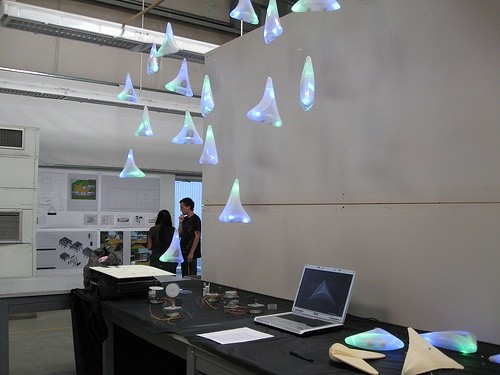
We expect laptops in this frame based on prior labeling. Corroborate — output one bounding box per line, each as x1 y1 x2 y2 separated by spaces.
254 265 356 337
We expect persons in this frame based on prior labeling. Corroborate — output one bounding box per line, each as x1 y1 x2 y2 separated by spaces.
177 197 202 276
146 209 180 278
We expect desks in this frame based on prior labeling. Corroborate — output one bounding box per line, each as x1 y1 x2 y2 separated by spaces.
70 279 500 375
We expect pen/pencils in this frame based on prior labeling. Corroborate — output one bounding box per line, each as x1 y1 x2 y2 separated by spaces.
289 351 314 362
